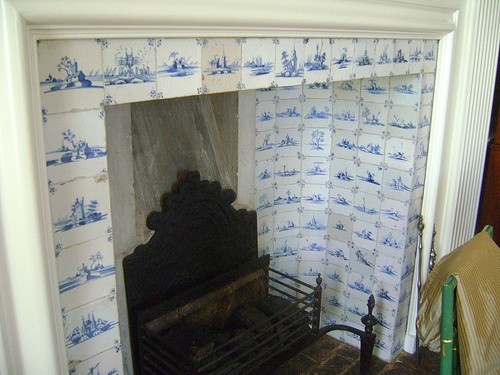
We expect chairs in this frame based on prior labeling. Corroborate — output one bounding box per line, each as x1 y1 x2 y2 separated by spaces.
438 224 500 375
